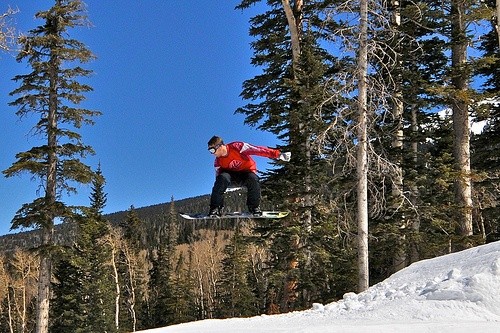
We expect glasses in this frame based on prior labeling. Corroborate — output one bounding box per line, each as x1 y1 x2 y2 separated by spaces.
208 144 222 154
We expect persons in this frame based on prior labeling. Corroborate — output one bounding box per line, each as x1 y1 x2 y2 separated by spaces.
206 135 290 216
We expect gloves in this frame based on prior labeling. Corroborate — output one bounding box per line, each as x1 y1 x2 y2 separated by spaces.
280 152 292 162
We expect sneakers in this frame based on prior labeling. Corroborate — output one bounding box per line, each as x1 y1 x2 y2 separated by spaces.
209 206 224 218
248 207 262 217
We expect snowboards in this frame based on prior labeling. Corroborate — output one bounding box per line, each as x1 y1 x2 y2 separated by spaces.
179 210 290 220
